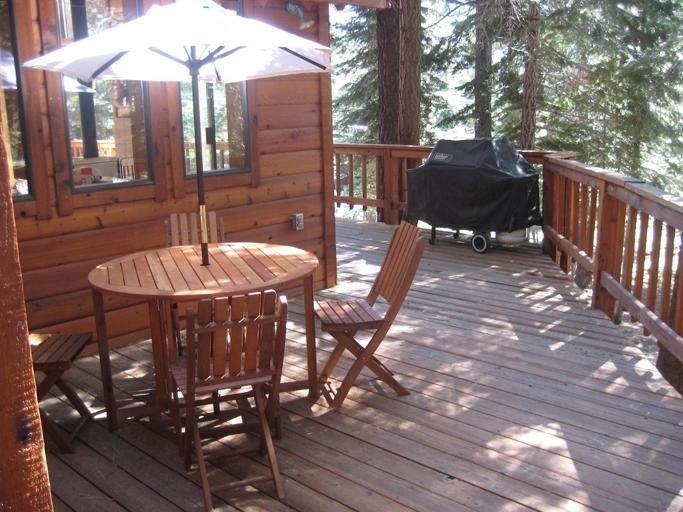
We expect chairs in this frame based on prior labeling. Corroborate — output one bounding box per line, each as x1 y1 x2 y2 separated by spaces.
165 288 289 512
163 211 225 359
312 220 425 408
29 332 93 453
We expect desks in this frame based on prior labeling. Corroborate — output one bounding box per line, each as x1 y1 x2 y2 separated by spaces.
89 240 319 431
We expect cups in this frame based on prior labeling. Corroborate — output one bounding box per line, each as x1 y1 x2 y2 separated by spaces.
78 174 92 186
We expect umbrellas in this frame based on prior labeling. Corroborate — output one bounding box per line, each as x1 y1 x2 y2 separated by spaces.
22 0 331 413
